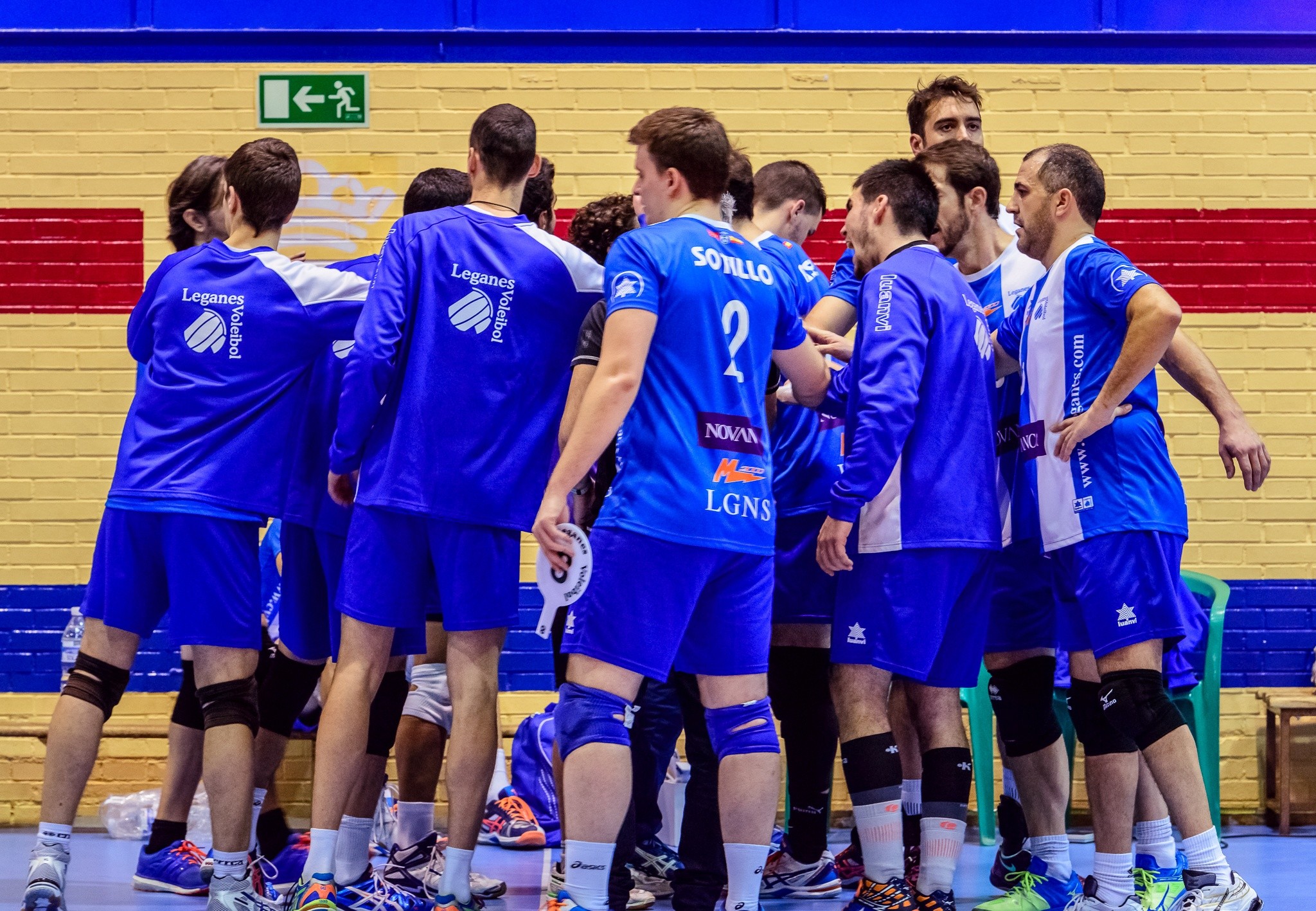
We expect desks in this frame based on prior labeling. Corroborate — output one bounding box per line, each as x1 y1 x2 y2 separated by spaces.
1265 697 1316 837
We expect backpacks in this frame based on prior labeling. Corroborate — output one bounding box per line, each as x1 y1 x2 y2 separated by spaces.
510 703 564 849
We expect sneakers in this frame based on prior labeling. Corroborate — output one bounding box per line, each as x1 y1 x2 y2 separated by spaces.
909 889 956 911
974 855 1085 911
842 878 919 911
723 846 842 897
383 831 507 898
630 836 686 880
1063 874 1140 911
20 842 70 911
132 840 208 896
206 874 276 911
549 860 657 909
1181 869 1265 910
262 831 311 895
1133 849 1188 911
478 785 548 848
630 866 672 897
834 842 920 885
989 845 1084 893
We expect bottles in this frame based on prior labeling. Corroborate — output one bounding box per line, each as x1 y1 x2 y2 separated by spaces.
61 607 85 693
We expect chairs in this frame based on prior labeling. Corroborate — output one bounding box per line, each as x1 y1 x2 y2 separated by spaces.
961 567 1231 851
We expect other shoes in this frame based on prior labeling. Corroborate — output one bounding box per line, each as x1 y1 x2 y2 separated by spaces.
435 893 485 911
540 889 584 910
335 863 434 911
288 873 337 911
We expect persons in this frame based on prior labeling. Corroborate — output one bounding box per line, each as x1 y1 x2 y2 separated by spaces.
27 76 1272 911
